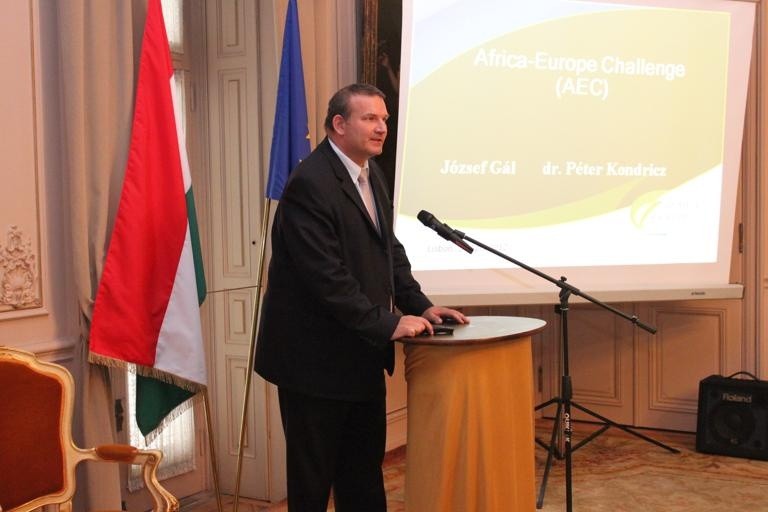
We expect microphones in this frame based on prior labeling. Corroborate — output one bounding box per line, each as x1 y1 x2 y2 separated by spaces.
417 210 473 254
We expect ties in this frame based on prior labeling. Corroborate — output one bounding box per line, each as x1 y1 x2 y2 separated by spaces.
357 167 382 233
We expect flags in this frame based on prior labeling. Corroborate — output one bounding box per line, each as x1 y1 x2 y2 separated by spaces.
88 0 207 447
267 0 311 200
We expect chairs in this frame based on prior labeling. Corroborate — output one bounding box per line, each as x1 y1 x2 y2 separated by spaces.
0 347 181 512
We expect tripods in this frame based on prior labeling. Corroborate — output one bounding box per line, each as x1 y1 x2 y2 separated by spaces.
436 224 680 512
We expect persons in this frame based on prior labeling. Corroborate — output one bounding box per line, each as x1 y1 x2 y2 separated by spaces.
253 85 469 512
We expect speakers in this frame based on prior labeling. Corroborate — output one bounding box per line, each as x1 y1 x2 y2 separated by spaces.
696 375 768 460
